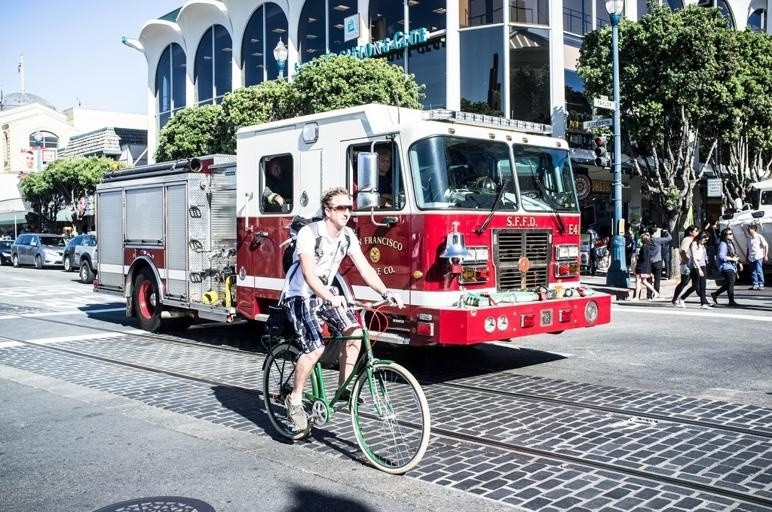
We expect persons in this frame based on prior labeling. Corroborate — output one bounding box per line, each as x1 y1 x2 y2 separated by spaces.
275 186 405 431
378 152 393 194
625 223 769 309
263 160 289 208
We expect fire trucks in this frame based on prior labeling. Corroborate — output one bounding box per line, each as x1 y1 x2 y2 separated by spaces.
91 102 614 348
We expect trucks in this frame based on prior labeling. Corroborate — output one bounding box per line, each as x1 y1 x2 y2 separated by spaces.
714 177 771 273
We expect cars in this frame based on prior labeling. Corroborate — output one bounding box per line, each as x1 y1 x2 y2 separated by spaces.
60 235 97 271
1 239 15 265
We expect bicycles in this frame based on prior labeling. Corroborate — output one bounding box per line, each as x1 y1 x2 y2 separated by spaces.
257 290 432 477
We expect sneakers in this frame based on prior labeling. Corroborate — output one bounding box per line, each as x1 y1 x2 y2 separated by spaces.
335 389 363 403
702 291 739 308
753 285 764 289
672 296 686 307
285 394 307 430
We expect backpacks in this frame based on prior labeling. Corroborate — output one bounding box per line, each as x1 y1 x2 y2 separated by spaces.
283 215 350 274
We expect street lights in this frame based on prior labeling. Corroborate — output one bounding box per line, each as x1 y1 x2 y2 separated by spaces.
35 131 44 174
272 36 287 80
601 0 632 287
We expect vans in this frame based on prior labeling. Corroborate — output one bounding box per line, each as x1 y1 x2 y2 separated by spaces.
10 233 67 269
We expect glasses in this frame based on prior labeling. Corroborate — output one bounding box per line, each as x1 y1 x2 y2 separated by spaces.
328 205 352 211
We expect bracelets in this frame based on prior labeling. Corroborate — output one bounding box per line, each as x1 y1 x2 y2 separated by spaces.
380 289 393 300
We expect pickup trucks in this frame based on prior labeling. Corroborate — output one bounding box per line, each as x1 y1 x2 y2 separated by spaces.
74 241 97 284
